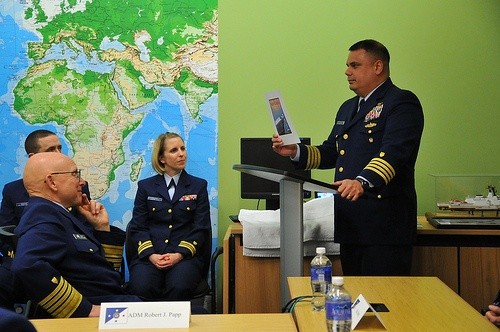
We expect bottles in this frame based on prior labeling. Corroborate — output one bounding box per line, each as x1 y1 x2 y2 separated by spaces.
310 247 332 312
324 276 352 332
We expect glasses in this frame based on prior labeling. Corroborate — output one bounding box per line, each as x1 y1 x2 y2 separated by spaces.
44 169 81 183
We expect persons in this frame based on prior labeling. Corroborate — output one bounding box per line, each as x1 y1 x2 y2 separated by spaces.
121 131 213 304
11 146 127 318
0 129 91 295
271 40 426 277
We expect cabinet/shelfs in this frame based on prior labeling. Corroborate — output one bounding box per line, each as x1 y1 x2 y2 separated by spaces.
236 235 500 313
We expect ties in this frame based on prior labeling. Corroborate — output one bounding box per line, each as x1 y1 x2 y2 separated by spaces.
359 98 365 112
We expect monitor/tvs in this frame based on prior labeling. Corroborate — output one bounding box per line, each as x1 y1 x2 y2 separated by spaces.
240 138 311 212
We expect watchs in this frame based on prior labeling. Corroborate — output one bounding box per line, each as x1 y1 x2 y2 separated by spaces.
356 177 370 195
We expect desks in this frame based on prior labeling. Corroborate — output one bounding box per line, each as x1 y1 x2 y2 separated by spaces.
30 313 298 332
287 276 500 332
